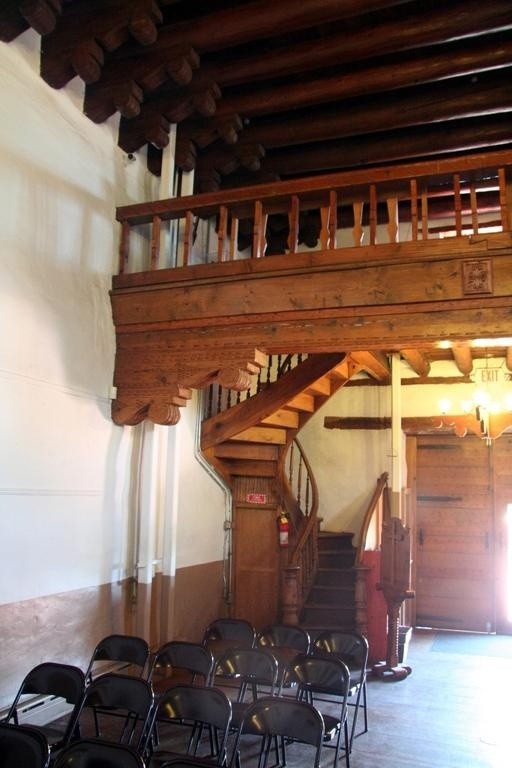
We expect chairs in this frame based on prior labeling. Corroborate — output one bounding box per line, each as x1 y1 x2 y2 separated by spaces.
0 616 369 768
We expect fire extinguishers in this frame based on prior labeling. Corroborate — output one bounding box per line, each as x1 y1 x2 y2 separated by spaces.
277 509 290 548
